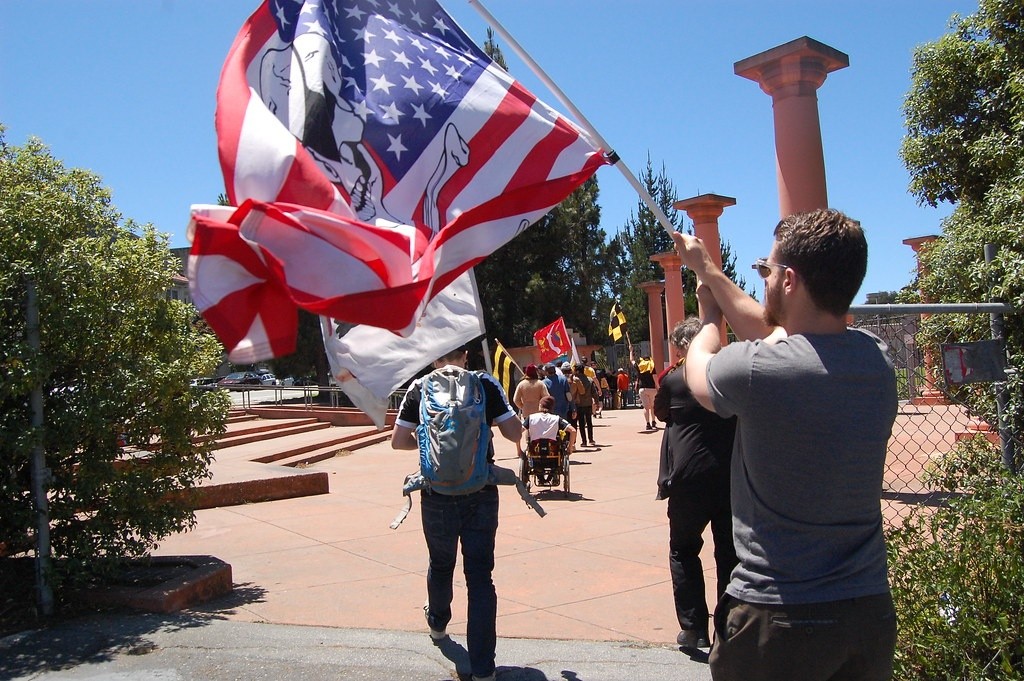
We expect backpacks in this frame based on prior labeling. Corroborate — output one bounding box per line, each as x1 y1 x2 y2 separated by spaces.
390 364 548 529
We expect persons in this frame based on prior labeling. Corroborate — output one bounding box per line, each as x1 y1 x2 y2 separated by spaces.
516 395 576 485
391 346 521 681
672 207 899 681
513 364 551 421
523 357 600 447
629 345 660 430
654 316 739 648
601 368 628 409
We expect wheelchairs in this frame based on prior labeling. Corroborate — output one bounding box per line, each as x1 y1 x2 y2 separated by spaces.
519 438 571 495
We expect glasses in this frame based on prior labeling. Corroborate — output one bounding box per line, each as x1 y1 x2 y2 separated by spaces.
756 257 805 282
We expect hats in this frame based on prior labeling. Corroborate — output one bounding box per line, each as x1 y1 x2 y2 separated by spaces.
539 396 555 410
526 363 536 377
561 361 571 371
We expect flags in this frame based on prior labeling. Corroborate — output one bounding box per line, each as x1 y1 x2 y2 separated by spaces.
187 1 612 363
492 345 519 414
534 316 571 364
319 268 487 432
609 301 629 342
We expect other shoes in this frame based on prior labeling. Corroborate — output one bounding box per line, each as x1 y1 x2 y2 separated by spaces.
552 479 559 485
538 479 547 485
580 441 587 446
651 421 657 429
677 628 711 648
589 440 595 444
424 605 446 639
646 422 652 430
472 671 496 681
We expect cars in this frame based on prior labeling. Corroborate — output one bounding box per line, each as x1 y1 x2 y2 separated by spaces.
188 376 225 389
259 374 276 386
275 377 295 387
217 372 263 392
